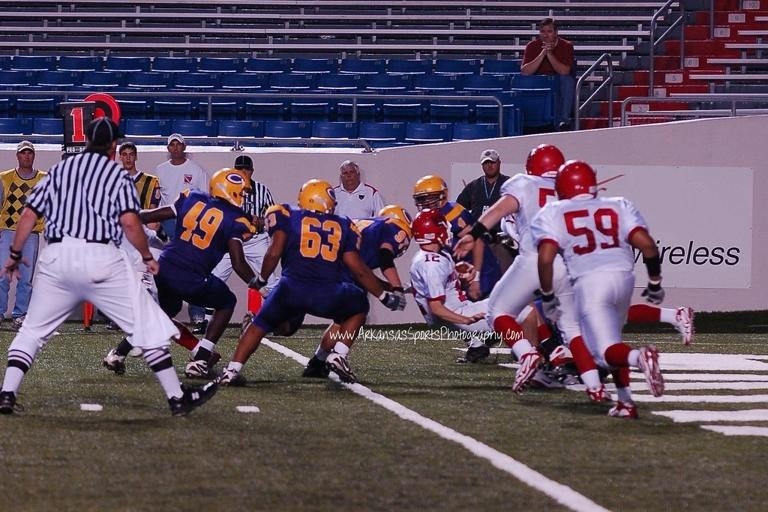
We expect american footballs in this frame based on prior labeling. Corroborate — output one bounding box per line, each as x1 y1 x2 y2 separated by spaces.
455 261 476 289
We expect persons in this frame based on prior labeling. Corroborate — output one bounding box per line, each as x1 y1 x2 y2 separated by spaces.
520 18 576 129
412 174 502 362
103 168 271 380
302 204 414 378
0 116 217 421
210 155 281 294
119 142 162 210
408 208 567 390
0 140 49 332
501 213 693 346
331 160 384 218
455 148 520 278
213 179 406 386
530 159 666 418
118 223 165 357
452 143 612 403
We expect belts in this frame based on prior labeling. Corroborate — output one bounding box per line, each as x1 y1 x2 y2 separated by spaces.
46 235 111 245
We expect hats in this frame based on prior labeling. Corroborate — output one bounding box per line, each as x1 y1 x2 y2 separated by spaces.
85 117 126 144
166 133 186 146
16 140 35 153
479 149 499 165
233 155 255 172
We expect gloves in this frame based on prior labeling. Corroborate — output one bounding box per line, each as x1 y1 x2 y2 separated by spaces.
640 277 666 306
389 287 407 311
539 289 563 323
248 275 268 292
378 290 400 312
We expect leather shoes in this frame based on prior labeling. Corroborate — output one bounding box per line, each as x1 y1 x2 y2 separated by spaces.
559 122 574 132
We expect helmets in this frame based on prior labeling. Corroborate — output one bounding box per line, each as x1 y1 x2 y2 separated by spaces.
524 143 565 179
554 159 598 201
409 208 454 249
207 167 253 209
297 178 338 215
378 204 413 239
411 174 449 213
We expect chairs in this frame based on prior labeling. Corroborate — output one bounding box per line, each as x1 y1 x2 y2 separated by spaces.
0 54 556 149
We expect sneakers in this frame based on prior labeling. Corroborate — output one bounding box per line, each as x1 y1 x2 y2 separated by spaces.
674 306 696 347
608 399 641 420
192 320 208 335
183 359 213 380
166 379 218 418
586 384 612 406
325 347 357 385
100 347 127 377
527 366 566 391
189 339 222 369
220 367 240 386
106 320 121 331
0 386 16 416
637 345 665 398
511 345 546 394
301 354 332 379
456 344 491 364
